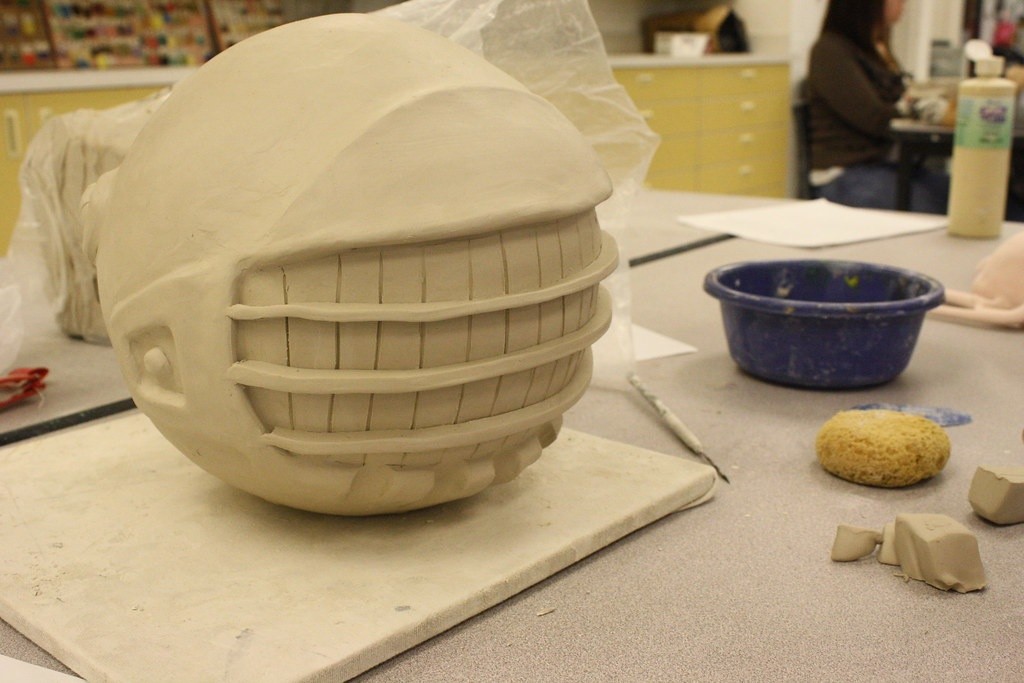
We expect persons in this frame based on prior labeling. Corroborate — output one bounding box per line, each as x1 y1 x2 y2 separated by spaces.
796 0 949 215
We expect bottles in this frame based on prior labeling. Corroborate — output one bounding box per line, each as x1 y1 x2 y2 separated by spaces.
945 38 1017 239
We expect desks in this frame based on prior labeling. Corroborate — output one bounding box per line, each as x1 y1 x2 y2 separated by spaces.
0 191 1024 683
891 120 1024 213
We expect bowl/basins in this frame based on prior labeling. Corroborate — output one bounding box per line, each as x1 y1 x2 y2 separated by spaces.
702 260 947 391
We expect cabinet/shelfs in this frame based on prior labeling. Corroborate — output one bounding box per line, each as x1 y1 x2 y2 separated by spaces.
616 53 794 200
0 65 195 254
